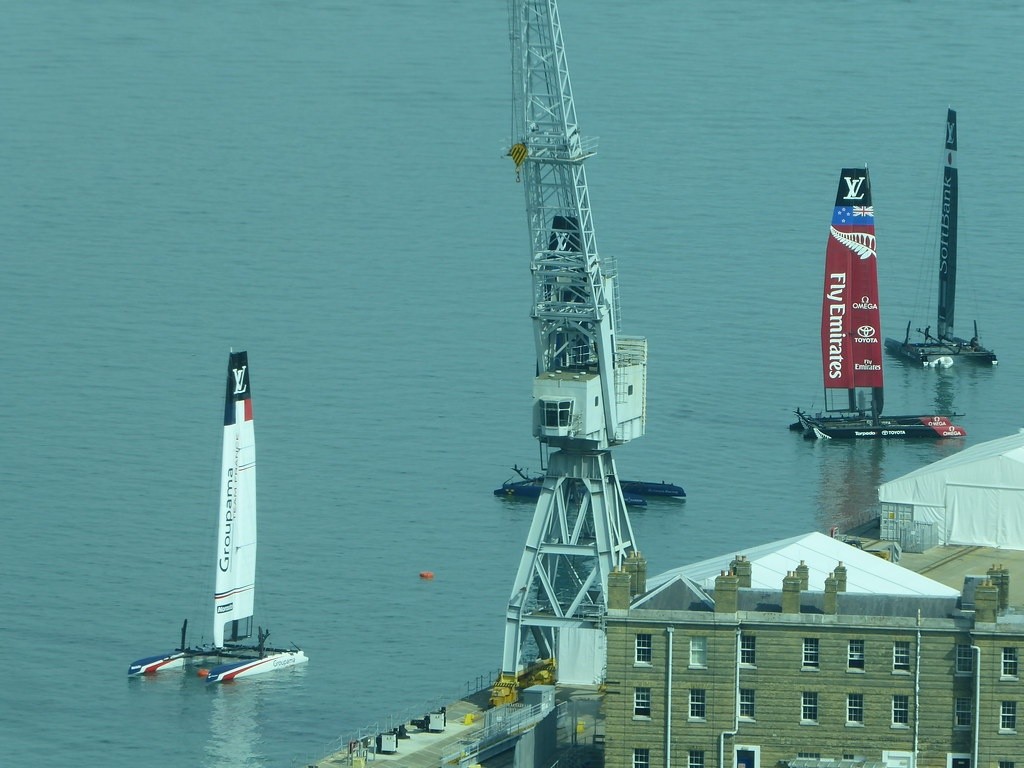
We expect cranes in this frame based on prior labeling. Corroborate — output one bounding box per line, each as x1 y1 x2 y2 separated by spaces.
497 0 650 447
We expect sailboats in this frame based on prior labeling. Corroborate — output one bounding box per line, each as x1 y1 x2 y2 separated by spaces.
494 215 688 508
128 343 309 684
786 162 967 440
884 105 1000 368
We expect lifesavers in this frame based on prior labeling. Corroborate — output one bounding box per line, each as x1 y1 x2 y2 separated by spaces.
198 668 209 676
